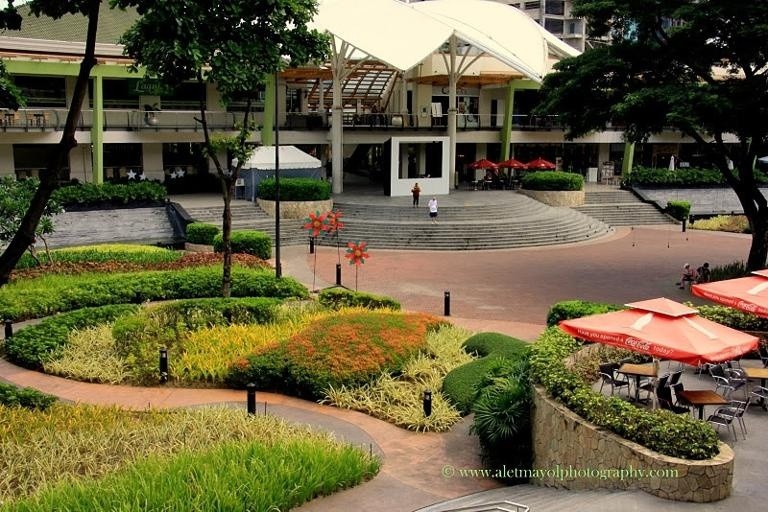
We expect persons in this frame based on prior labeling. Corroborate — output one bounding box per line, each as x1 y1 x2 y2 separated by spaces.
371 105 377 126
427 196 440 225
695 262 711 283
411 182 421 208
675 262 699 289
379 105 385 126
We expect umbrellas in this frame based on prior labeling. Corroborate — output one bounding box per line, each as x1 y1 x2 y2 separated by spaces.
689 268 768 322
551 293 760 408
469 156 557 187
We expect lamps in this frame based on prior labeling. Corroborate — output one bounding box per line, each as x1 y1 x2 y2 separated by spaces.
391 113 406 130
145 110 160 126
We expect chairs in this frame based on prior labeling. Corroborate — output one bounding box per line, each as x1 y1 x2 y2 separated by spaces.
696 345 767 411
706 401 741 449
597 362 631 399
653 369 684 388
656 398 671 411
656 386 673 406
634 374 668 406
464 168 521 193
715 395 750 440
672 382 692 409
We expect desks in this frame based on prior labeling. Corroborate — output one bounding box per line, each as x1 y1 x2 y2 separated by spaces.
679 390 730 421
617 363 656 406
